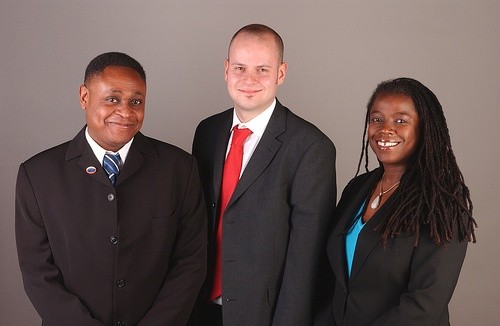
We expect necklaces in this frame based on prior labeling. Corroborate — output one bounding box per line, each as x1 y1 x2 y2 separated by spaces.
371 172 400 209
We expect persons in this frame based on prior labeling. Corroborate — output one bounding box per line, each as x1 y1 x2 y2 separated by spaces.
15 51 209 326
312 77 478 326
191 23 337 326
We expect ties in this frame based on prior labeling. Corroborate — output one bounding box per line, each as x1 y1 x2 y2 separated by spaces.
209 124 252 301
103 152 123 186
